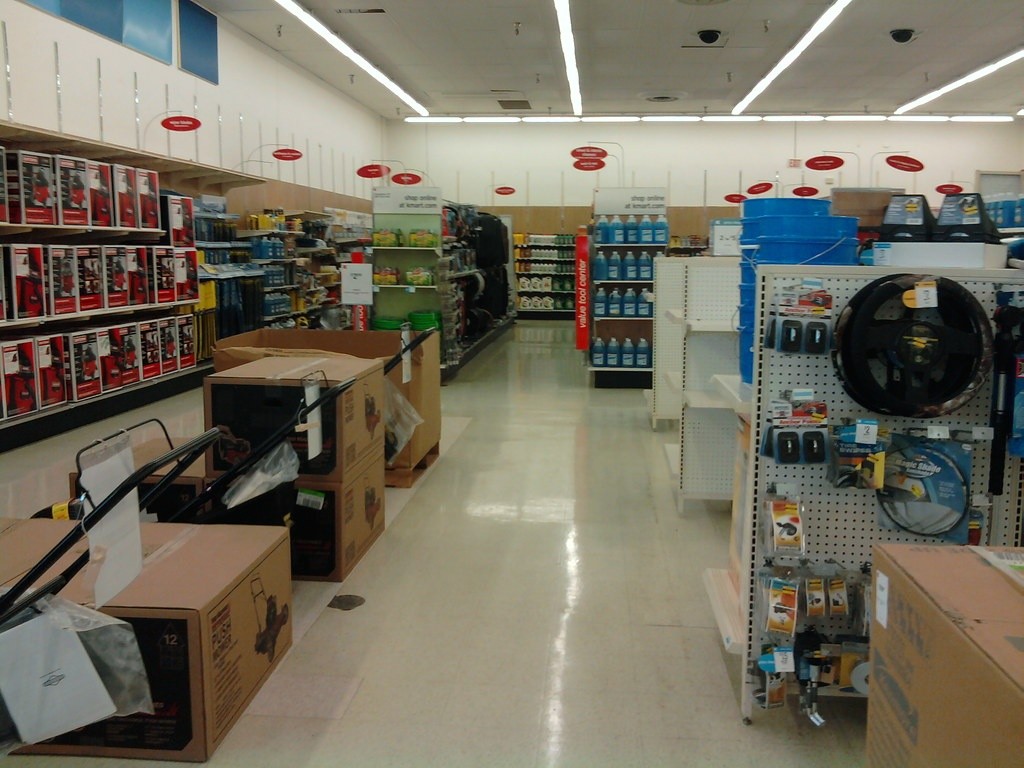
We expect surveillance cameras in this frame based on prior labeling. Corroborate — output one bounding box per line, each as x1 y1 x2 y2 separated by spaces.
698 30 721 44
889 29 915 43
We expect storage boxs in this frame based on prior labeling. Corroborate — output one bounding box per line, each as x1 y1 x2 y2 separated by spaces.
0 145 199 421
211 328 441 468
0 516 293 763
69 357 385 581
864 545 1024 768
709 218 743 256
246 215 272 229
200 195 226 213
873 241 1009 269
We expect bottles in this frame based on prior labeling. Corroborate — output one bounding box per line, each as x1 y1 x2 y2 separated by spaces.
595 215 608 243
639 251 653 281
519 276 577 291
252 237 285 259
623 251 637 280
594 287 653 317
271 318 296 330
669 235 709 256
406 266 436 285
204 250 250 265
610 215 625 244
626 215 638 244
981 192 1024 228
520 295 575 310
196 219 237 242
289 290 306 310
593 337 651 368
656 251 665 256
638 215 653 243
373 228 439 247
262 208 285 231
373 267 400 285
608 251 622 281
594 251 608 281
263 265 290 287
654 215 669 244
264 294 291 315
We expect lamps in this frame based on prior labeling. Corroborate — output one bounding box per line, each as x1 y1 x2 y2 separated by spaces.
274 0 429 116
894 45 1024 115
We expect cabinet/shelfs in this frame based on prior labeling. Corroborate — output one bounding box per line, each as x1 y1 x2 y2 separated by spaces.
370 187 517 385
585 244 667 388
703 264 1024 726
0 221 359 455
664 257 741 515
514 243 709 320
